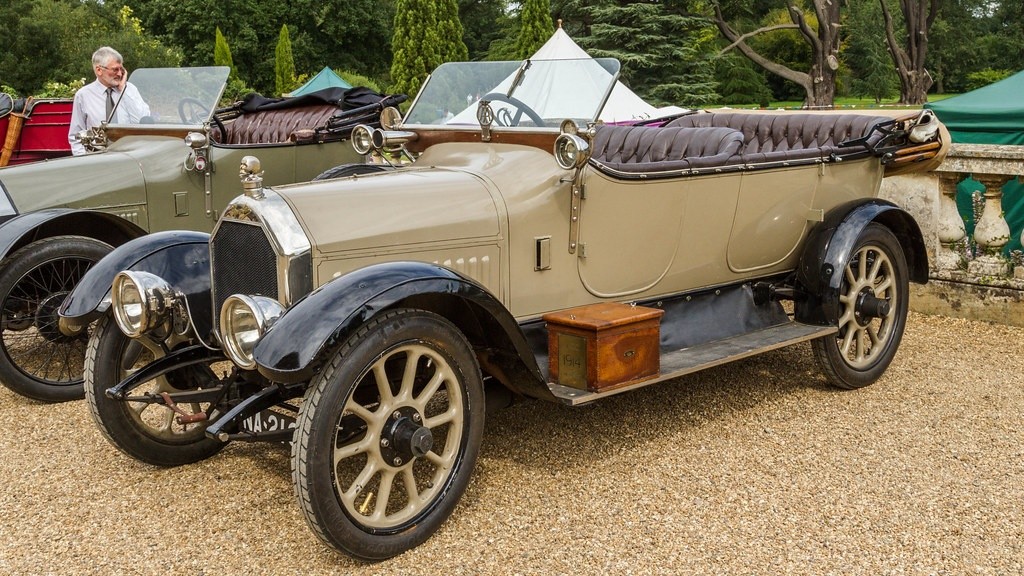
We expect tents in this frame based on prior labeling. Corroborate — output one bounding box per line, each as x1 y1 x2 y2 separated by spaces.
281 64 354 100
447 19 664 125
922 69 1024 145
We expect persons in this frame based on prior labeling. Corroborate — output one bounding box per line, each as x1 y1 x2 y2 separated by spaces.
467 92 473 106
442 108 454 124
68 46 151 156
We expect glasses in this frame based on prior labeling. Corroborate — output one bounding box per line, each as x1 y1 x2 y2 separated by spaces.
96 65 124 72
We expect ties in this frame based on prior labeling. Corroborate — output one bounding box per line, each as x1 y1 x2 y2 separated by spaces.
105 88 118 124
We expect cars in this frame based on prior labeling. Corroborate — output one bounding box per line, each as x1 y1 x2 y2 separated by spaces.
0 66 417 405
57 55 951 564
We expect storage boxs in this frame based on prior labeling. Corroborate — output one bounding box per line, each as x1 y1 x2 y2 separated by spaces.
542 301 665 394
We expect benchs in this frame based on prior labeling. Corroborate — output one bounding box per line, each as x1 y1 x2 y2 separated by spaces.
208 102 369 145
583 113 896 172
